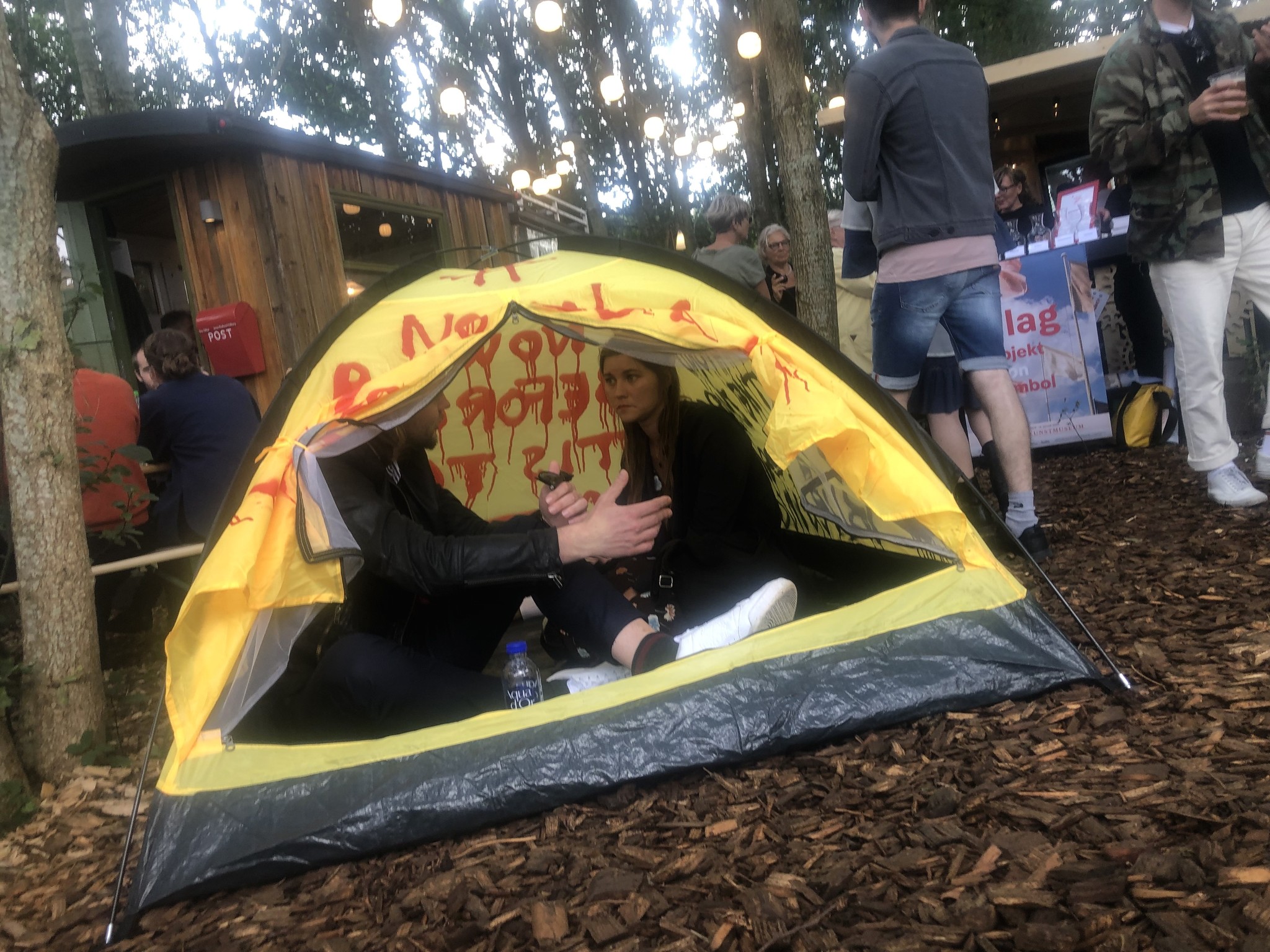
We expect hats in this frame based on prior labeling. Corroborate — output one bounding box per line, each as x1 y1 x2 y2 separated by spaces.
828 209 843 228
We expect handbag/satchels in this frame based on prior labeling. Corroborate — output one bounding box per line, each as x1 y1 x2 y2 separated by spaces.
1108 381 1178 451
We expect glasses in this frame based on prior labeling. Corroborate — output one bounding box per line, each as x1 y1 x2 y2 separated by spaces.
744 215 753 225
999 184 1014 196
768 239 791 252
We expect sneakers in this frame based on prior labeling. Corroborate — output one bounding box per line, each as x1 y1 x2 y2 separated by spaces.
673 577 798 663
1254 435 1270 479
1206 464 1268 506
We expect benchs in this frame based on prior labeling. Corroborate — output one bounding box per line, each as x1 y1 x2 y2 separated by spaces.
0 543 204 595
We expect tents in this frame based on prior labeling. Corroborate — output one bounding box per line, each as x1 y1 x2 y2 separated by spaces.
104 235 1133 945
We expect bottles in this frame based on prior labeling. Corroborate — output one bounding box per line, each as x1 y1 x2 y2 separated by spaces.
1003 219 1026 259
500 642 544 709
1076 202 1102 244
1050 207 1076 250
1027 212 1051 254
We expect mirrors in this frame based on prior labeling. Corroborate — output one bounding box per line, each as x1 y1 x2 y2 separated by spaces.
1038 148 1119 228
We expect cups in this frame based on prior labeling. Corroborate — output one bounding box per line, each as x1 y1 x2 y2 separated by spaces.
1208 65 1250 118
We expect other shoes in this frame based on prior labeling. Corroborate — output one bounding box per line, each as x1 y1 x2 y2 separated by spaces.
995 521 1055 564
966 500 993 528
100 570 162 670
988 463 1010 521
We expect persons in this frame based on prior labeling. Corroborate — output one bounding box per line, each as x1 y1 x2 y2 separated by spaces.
840 0 1048 555
75 331 262 631
690 192 772 303
229 388 797 738
994 166 1054 245
840 173 1019 519
759 224 797 318
512 337 784 665
1089 0 1270 506
1089 172 1163 387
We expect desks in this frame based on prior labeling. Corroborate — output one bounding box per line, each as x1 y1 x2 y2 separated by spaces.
963 232 1178 458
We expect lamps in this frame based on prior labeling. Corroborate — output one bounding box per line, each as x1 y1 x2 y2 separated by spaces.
199 195 222 224
